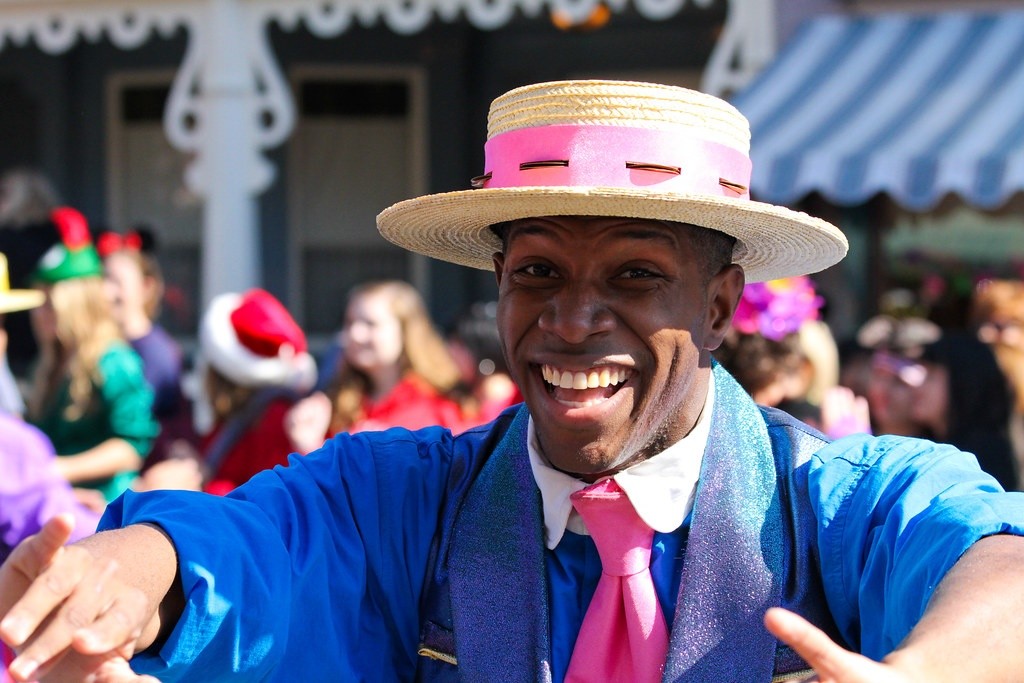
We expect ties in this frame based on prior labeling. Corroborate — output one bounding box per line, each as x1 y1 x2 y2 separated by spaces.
563 478 669 683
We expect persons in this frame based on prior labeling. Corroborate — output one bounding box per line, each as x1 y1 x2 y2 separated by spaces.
718 266 1024 493
0 209 524 683
0 79 1024 683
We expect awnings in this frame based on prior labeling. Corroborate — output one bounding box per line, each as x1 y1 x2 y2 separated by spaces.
719 11 1024 209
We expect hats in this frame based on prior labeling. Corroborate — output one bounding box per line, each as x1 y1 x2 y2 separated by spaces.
35 207 101 283
0 252 45 315
204 289 317 394
376 80 849 283
